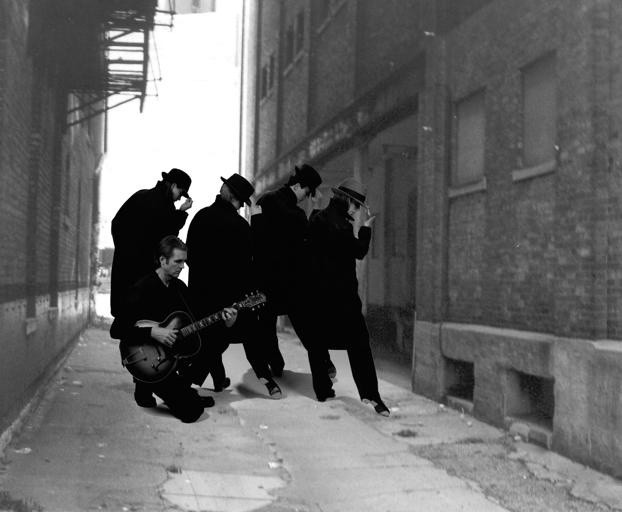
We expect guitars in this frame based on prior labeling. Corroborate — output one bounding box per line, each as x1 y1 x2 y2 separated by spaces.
118 288 267 384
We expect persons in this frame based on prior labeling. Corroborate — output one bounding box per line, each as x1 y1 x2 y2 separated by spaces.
110 234 239 423
248 163 338 379
108 166 193 317
184 172 284 400
303 178 391 417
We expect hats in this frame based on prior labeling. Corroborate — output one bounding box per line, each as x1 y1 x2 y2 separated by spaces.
162 169 193 199
331 177 367 206
295 164 322 196
220 173 254 205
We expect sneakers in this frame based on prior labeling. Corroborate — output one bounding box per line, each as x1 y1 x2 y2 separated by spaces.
216 377 230 391
134 384 157 407
319 368 336 402
200 396 215 408
257 371 281 399
361 394 391 418
